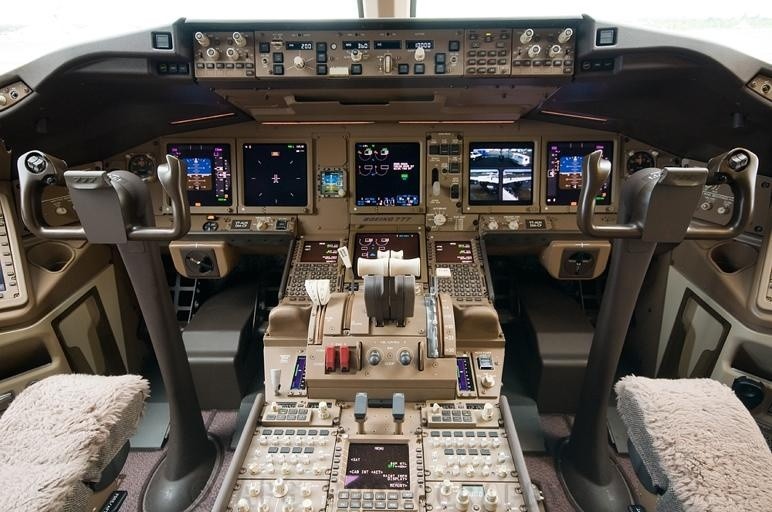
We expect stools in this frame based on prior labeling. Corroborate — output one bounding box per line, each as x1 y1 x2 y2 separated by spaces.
0 372 150 512
614 374 772 512
